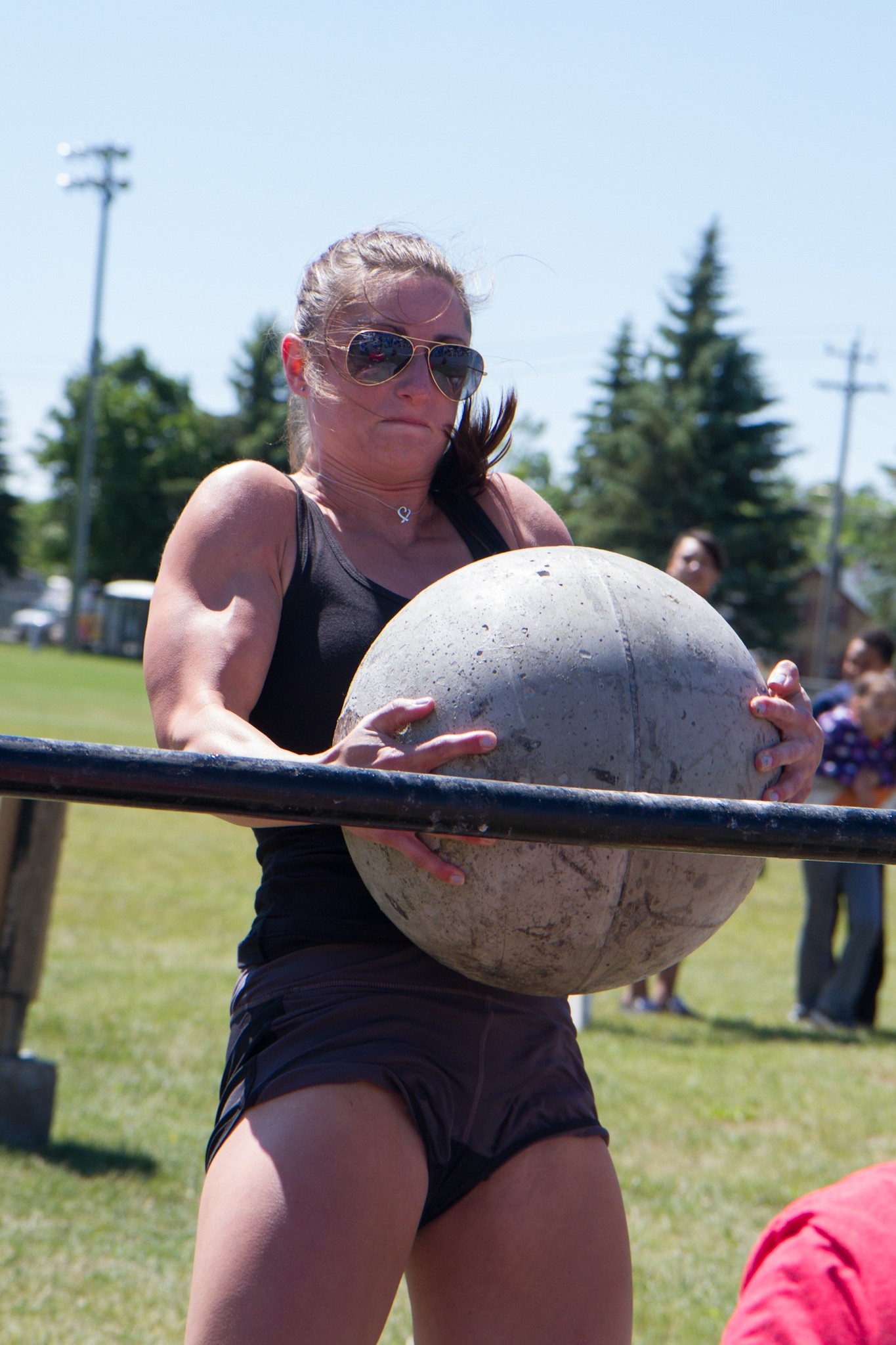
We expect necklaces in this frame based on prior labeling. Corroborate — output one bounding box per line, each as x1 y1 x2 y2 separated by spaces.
305 464 428 524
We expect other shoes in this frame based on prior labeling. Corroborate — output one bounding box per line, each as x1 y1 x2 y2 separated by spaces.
623 996 659 1012
661 996 687 1014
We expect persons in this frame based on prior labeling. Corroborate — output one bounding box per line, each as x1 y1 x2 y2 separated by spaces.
623 527 726 1017
721 1161 896 1345
793 630 896 1038
144 228 826 1345
356 346 385 371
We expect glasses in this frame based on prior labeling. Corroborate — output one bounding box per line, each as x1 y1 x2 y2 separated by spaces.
300 330 486 403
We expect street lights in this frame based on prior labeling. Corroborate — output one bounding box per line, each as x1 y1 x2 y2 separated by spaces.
53 136 132 654
